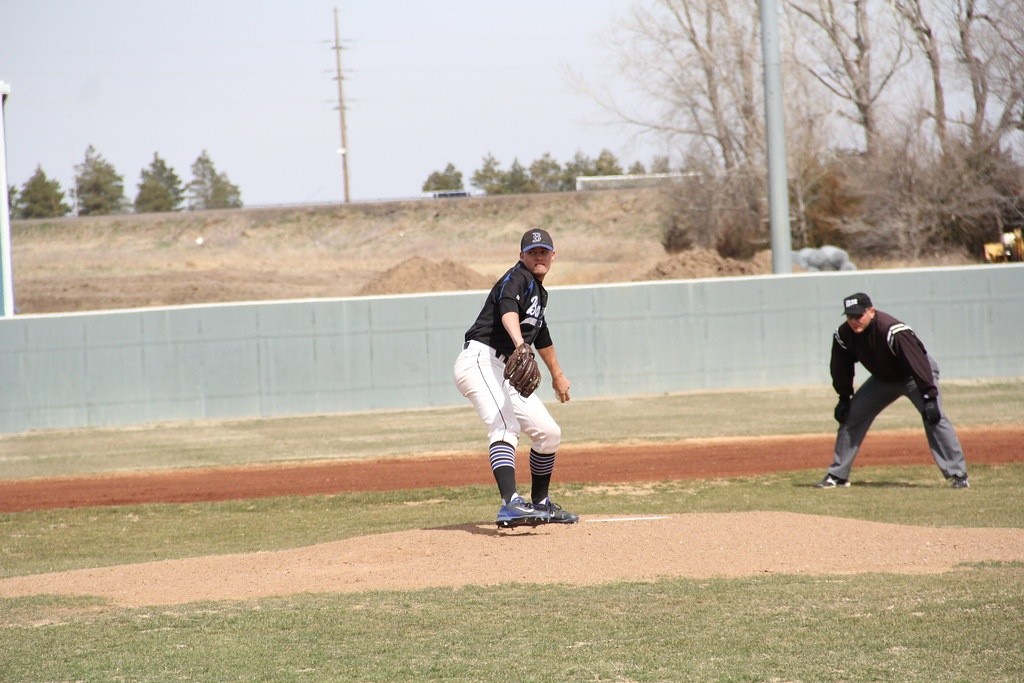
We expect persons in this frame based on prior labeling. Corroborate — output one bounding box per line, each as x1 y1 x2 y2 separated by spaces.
454 228 578 528
818 292 970 489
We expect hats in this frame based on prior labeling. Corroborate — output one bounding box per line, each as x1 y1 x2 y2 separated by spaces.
841 292 872 316
520 228 553 252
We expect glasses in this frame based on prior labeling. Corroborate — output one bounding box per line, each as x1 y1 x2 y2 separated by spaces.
845 313 863 321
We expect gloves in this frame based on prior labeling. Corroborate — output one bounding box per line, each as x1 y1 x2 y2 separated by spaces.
924 398 941 425
834 396 850 423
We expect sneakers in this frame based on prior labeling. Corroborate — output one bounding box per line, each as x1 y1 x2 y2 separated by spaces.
532 497 579 525
950 473 969 488
816 474 850 490
495 497 551 528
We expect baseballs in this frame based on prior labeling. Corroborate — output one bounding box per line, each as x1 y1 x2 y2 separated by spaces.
555 392 563 401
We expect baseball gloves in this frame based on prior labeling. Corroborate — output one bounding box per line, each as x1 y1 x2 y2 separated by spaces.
503 343 543 398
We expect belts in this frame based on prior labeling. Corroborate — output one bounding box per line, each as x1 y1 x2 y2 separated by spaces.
463 342 510 363
